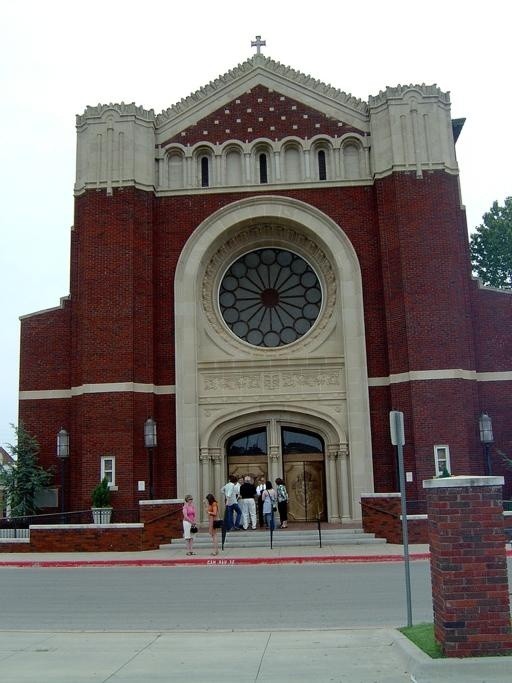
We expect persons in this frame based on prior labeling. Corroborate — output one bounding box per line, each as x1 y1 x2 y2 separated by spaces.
206 494 219 556
221 475 289 532
182 495 197 555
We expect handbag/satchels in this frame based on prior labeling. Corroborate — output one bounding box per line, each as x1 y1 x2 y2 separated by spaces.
272 500 277 512
191 524 198 533
214 520 224 528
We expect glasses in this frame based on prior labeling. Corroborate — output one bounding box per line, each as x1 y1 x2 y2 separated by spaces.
189 498 193 501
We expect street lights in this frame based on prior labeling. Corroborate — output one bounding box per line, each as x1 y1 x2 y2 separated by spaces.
56 426 70 524
478 414 495 476
143 419 158 500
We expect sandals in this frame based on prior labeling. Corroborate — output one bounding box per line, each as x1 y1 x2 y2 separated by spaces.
280 525 287 528
185 552 196 556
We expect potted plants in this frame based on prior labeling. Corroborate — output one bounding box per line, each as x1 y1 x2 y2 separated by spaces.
90 475 114 524
